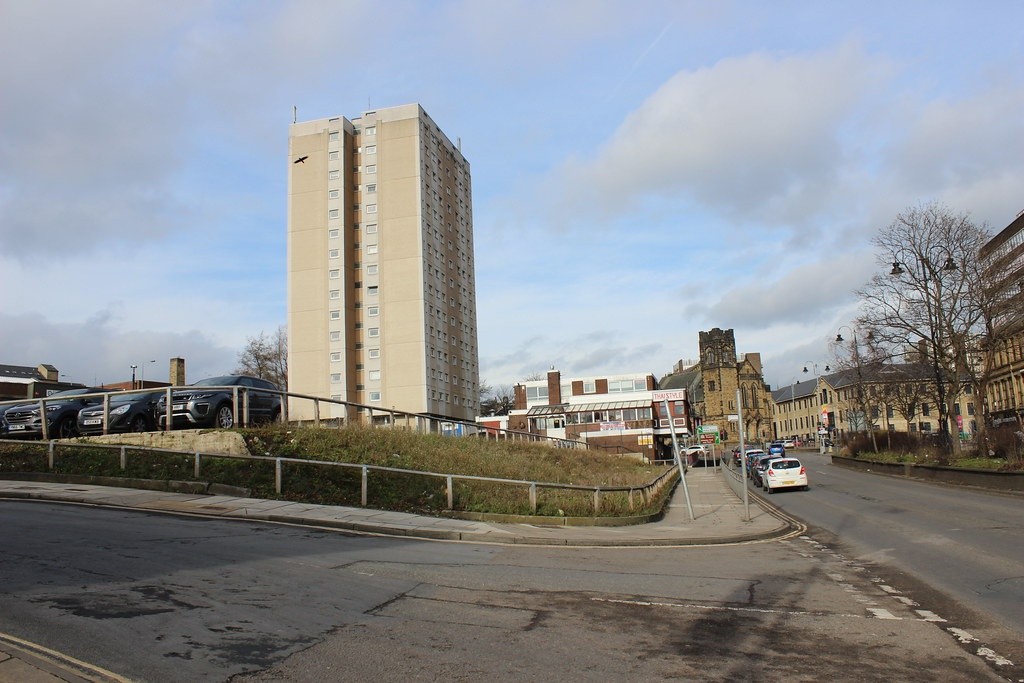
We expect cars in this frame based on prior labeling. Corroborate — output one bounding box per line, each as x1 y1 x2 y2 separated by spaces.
76 388 168 436
824 438 834 447
731 445 783 487
766 444 785 458
763 458 808 493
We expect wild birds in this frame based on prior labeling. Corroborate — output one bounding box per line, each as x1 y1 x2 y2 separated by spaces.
294 156 308 164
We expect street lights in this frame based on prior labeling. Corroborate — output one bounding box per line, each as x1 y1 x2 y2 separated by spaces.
791 374 800 412
836 326 876 386
130 365 137 390
802 358 830 427
141 360 156 389
891 243 959 415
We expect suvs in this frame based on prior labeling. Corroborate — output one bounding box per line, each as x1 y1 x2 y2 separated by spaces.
153 375 284 430
773 439 795 449
0 386 123 440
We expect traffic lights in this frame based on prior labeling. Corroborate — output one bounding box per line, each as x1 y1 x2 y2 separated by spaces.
722 430 728 441
